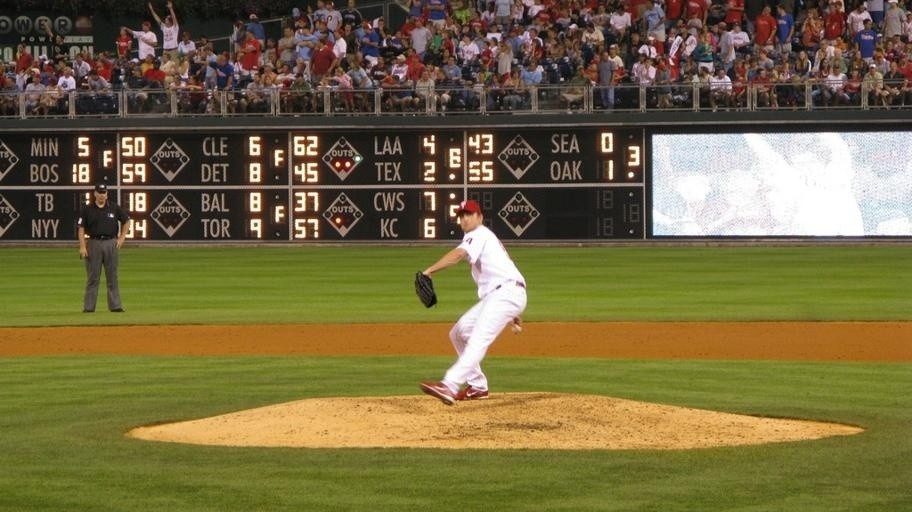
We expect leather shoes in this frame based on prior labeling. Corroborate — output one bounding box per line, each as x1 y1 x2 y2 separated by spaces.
82 309 95 313
111 308 125 313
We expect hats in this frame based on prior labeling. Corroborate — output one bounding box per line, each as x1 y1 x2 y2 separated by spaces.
887 0 898 4
869 64 876 68
456 200 481 215
95 183 107 193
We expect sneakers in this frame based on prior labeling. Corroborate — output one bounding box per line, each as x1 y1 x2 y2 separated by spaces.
455 386 490 400
419 381 455 404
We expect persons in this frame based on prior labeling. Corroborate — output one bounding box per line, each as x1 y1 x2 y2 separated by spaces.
78 181 130 312
418 200 528 406
0 0 912 113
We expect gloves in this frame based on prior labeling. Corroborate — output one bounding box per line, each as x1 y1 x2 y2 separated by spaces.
512 317 524 335
89 235 115 240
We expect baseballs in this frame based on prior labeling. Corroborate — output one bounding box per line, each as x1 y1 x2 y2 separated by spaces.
512 324 522 335
